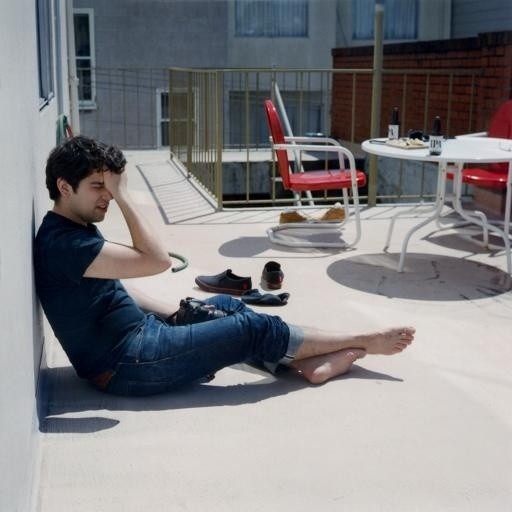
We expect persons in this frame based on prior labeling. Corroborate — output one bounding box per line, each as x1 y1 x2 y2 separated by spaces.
33 134 415 397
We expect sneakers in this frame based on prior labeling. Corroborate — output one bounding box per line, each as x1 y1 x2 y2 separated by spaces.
279 207 346 224
195 269 252 296
261 261 284 289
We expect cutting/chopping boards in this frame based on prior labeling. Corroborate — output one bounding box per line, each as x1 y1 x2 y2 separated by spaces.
387 144 429 150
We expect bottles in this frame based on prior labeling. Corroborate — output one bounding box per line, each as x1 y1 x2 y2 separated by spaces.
387 106 400 139
429 115 443 155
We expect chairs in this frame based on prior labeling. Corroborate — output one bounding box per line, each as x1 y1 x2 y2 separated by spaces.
435 100 512 240
264 100 367 248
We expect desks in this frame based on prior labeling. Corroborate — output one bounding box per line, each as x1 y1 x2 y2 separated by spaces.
361 135 512 274
